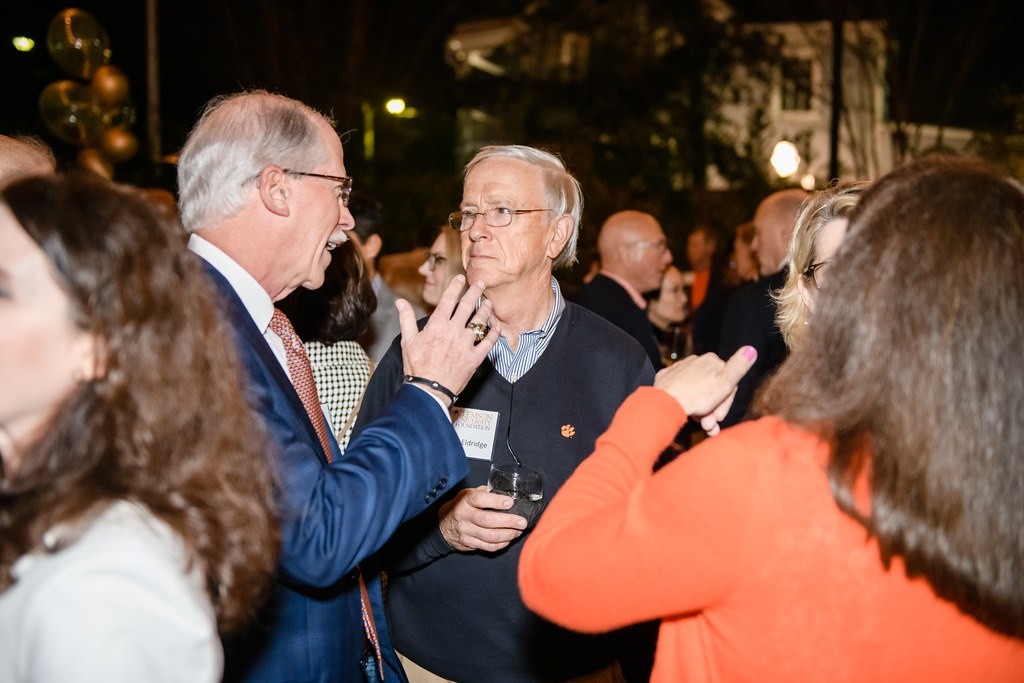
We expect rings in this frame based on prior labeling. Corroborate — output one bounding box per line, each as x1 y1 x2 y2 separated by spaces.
467 322 485 340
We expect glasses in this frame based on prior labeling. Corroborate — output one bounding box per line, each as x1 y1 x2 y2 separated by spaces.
448 208 554 232
425 253 447 271
283 168 353 206
627 241 668 253
803 262 831 291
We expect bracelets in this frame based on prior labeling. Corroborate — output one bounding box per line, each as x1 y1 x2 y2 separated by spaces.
400 373 459 411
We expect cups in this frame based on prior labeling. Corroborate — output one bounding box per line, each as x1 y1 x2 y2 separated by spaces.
486 463 544 545
663 327 692 363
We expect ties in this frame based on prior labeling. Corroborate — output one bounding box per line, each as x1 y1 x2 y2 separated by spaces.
268 307 384 680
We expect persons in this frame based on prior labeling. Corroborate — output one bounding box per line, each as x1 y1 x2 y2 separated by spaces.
272 229 380 458
343 197 429 366
422 223 480 308
173 89 504 682
0 130 58 183
513 152 1023 682
344 145 657 682
561 177 874 478
0 175 282 683
